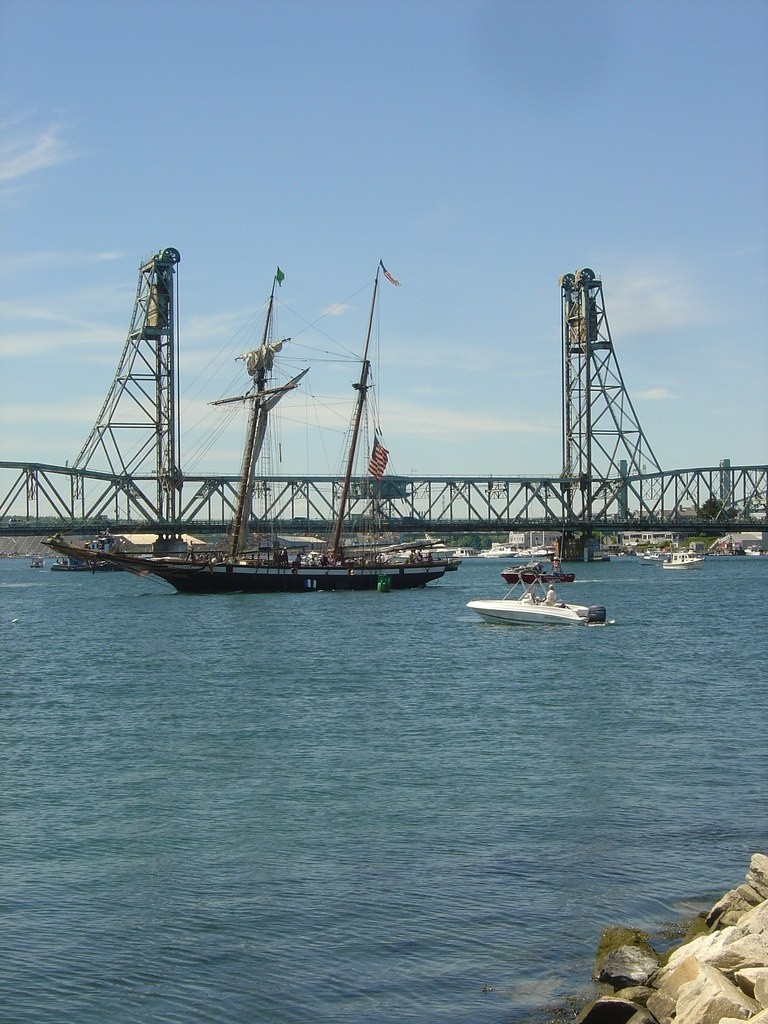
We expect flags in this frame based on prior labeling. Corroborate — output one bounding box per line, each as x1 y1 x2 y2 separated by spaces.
379 257 400 287
367 434 390 482
275 265 285 287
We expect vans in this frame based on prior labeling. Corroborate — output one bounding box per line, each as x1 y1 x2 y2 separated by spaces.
8 519 29 528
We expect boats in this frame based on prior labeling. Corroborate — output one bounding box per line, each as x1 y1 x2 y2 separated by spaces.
502 568 577 583
30 555 46 568
464 572 608 626
663 552 709 570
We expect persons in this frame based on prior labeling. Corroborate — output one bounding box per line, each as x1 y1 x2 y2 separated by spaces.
552 557 559 568
203 552 208 562
295 553 301 567
186 540 195 563
8 517 11 528
540 584 556 605
409 549 433 564
273 547 288 569
305 557 329 568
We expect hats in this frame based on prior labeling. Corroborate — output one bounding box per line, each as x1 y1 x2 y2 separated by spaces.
549 584 553 588
284 547 286 549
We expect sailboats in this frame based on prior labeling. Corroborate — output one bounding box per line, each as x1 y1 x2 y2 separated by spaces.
37 253 560 593
